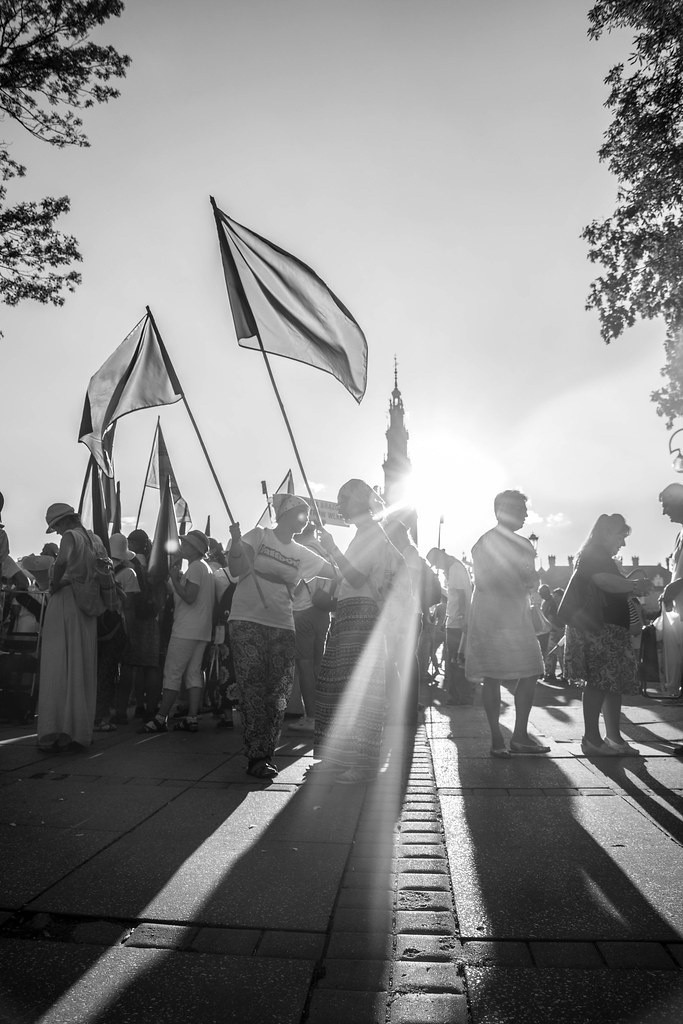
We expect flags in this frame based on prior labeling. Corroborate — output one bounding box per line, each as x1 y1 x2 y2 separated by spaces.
216 209 368 405
146 427 193 535
79 315 182 478
147 481 182 586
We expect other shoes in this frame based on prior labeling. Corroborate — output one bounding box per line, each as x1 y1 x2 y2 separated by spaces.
445 698 473 708
40 739 83 753
287 715 315 729
285 712 304 719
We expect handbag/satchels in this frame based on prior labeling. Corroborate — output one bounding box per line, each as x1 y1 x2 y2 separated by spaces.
557 553 609 632
311 589 338 611
653 600 683 699
531 604 552 636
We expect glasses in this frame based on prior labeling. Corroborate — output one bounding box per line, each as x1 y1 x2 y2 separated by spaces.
335 499 347 509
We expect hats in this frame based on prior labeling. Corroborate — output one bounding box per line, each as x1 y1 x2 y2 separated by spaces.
179 529 210 559
129 529 150 543
46 543 60 556
387 509 417 543
109 533 137 560
273 493 309 522
45 503 81 533
340 479 387 511
426 548 445 568
207 537 223 558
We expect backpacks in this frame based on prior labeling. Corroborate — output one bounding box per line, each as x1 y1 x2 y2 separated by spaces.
72 532 118 617
113 556 169 622
217 568 238 623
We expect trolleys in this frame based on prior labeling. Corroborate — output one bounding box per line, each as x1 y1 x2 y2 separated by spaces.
0 586 51 725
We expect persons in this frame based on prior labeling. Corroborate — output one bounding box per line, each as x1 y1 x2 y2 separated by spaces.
227 493 339 780
659 484 683 755
562 514 650 757
471 491 551 758
307 480 394 784
0 494 683 751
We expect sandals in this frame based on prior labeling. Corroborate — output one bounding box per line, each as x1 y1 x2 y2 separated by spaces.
245 756 278 780
93 705 234 734
490 737 550 756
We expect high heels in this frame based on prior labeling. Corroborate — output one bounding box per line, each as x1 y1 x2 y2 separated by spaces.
581 735 639 757
